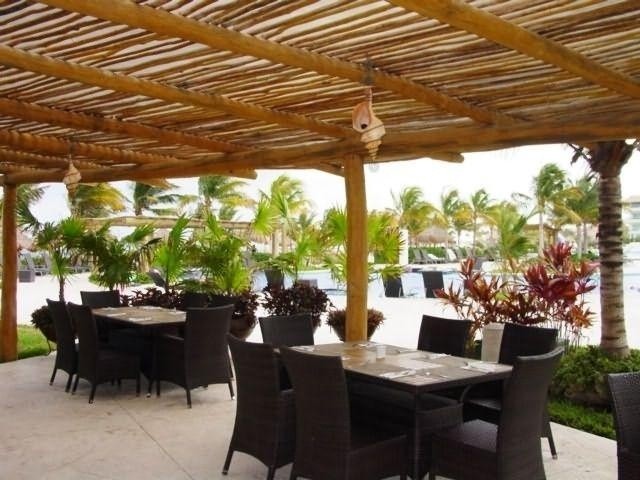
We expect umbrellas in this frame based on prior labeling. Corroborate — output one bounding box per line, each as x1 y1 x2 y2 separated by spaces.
414 226 458 255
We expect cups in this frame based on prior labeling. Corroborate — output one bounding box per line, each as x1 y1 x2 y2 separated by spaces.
375 346 386 359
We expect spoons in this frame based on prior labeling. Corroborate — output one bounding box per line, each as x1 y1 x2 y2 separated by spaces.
395 349 419 353
388 370 409 378
424 371 451 379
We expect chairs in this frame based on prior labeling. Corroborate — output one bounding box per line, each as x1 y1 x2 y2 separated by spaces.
45 290 236 409
609 368 640 480
